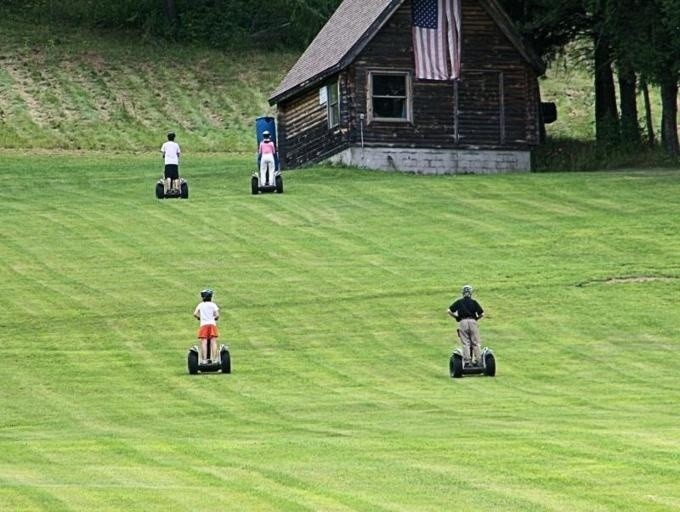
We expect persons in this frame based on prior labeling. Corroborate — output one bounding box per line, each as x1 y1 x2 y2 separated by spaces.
192 289 222 365
258 130 277 187
160 132 181 192
446 284 485 369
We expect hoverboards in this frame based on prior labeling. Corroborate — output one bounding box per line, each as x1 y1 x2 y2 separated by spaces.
250 173 285 195
155 175 191 201
184 338 233 377
447 340 499 379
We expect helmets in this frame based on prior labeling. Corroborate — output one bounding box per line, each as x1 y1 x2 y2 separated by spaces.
199 288 214 299
462 285 473 294
261 129 272 138
166 130 176 138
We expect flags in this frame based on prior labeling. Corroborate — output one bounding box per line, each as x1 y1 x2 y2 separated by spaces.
408 0 464 84
